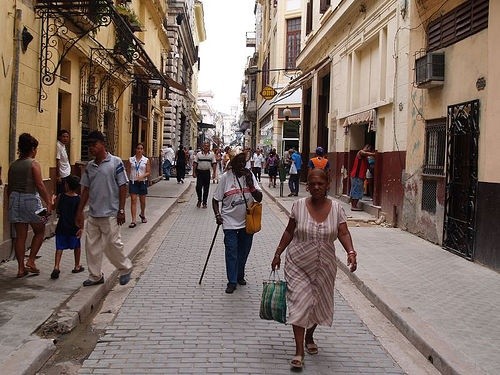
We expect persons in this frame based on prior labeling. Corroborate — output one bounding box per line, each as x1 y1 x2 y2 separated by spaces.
350 143 375 211
212 149 263 293
174 145 186 184
271 169 358 367
50 174 84 279
183 146 280 188
283 147 303 197
127 143 151 228
74 130 134 286
55 130 72 218
192 141 217 208
7 132 53 278
306 147 331 192
159 145 175 180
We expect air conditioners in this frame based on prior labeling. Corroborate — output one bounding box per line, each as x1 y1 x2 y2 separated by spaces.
415 53 445 85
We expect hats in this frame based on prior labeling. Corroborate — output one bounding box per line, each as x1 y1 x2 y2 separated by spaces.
226 146 252 170
80 131 104 144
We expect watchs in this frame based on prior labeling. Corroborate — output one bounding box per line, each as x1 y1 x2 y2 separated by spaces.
118 208 125 214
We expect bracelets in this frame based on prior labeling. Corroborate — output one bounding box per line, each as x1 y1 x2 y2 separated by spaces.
348 250 357 256
250 185 256 193
277 247 282 251
215 213 221 218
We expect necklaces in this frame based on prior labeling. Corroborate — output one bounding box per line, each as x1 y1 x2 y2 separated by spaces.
233 172 245 203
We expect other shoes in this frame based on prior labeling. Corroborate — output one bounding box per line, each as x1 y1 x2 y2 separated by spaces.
226 282 237 293
236 277 247 285
197 201 201 208
288 191 296 197
119 272 130 285
294 192 298 196
203 204 207 208
83 273 104 286
351 208 363 211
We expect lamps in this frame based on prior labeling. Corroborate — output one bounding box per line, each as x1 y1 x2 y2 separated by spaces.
249 69 301 74
283 106 291 121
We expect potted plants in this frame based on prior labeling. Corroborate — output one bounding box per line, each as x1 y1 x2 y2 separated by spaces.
115 3 147 32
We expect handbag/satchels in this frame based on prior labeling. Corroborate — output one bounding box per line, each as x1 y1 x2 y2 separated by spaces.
260 269 288 325
289 161 297 174
246 202 262 233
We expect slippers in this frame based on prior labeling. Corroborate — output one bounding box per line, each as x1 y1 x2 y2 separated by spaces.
304 342 318 354
24 266 40 273
129 223 136 228
139 214 147 223
290 356 304 368
72 266 85 274
51 270 60 280
17 267 29 278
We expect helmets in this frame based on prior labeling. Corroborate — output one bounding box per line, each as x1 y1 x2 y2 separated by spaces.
315 147 325 154
